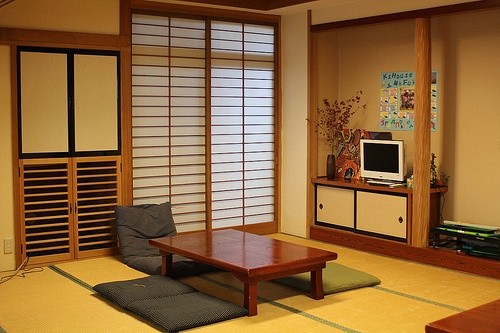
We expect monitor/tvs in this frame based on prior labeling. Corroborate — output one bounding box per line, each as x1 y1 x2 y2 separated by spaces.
359 139 405 185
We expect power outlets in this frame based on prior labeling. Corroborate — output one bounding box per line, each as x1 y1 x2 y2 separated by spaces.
4 238 13 254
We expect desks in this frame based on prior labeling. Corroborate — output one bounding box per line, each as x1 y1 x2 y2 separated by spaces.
150 228 338 317
424 299 500 333
433 226 500 256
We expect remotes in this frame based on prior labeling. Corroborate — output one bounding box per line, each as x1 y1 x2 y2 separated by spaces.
389 183 402 188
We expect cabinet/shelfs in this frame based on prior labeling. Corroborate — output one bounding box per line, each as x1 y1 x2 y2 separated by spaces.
311 176 448 244
14 45 134 268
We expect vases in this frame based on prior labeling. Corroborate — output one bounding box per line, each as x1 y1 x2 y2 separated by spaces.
326 154 335 181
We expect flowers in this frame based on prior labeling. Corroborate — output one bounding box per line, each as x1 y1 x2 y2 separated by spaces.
308 90 366 154
